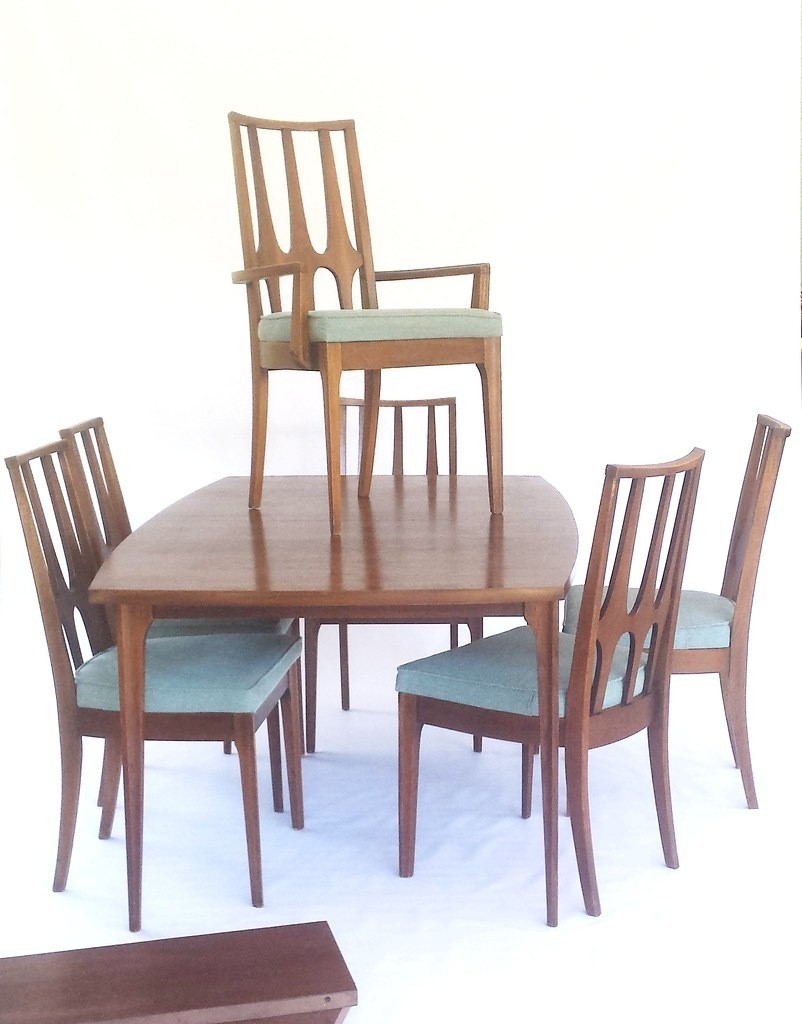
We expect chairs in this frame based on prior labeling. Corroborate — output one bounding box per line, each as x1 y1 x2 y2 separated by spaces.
59 415 307 813
226 112 504 538
396 446 705 918
564 412 793 811
4 439 307 933
339 396 484 754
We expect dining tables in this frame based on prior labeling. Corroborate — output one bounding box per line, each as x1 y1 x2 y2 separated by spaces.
89 473 578 930
